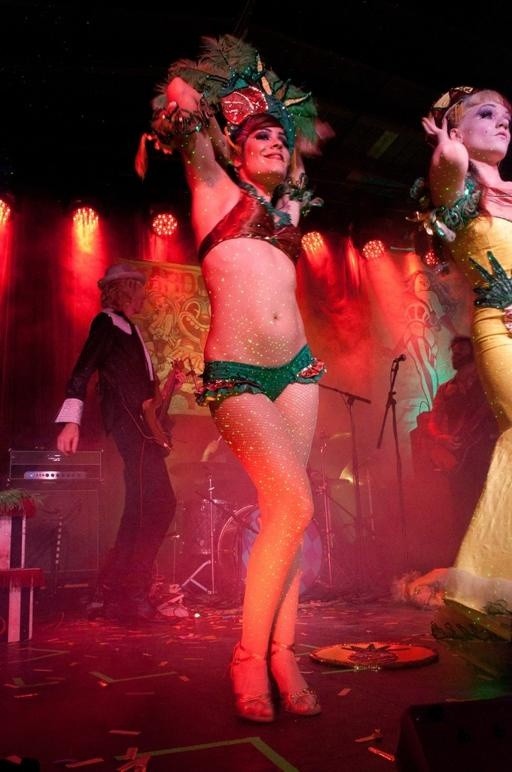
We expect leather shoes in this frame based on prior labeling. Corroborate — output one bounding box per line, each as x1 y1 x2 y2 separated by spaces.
260 640 324 716
225 639 279 728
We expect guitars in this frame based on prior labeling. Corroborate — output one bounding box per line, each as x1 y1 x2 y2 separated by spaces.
143 359 185 457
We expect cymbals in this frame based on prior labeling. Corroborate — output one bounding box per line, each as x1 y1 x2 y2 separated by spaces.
169 463 235 475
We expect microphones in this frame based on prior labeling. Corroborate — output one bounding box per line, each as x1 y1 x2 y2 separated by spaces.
392 354 406 362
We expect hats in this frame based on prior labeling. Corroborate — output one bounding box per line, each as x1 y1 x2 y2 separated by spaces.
92 259 148 286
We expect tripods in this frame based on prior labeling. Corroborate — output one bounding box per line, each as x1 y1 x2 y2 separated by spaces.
158 382 393 604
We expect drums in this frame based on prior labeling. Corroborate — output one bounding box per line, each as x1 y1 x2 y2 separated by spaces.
185 498 232 554
217 505 324 599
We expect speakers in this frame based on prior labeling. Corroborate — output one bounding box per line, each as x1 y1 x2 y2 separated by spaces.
10 481 99 588
399 696 512 772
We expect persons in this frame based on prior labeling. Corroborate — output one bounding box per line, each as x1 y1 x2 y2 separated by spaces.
406 81 512 699
151 73 334 726
52 262 187 627
